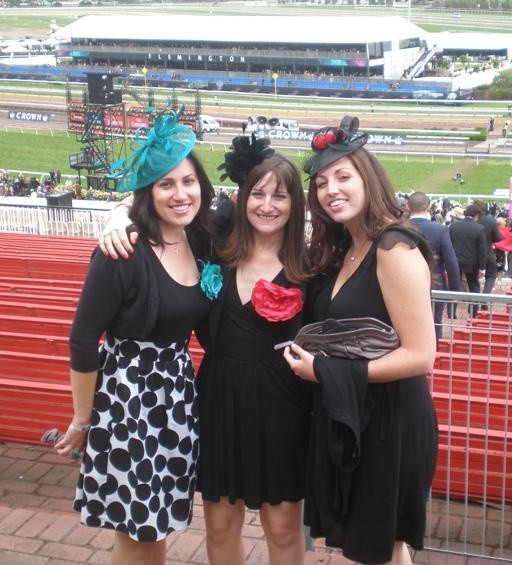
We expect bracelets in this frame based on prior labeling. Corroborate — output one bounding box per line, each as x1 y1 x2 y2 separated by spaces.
68 421 93 432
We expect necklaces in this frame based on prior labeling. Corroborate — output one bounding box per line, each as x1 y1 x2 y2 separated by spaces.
343 234 373 271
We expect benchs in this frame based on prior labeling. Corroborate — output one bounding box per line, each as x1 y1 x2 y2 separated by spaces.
0 232 512 504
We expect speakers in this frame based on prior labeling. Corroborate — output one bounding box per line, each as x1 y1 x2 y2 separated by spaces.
88 73 122 104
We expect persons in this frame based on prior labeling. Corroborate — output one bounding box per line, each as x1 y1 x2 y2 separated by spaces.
96 134 317 564
53 104 216 565
394 189 512 353
283 116 441 565
68 38 512 95
0 167 238 207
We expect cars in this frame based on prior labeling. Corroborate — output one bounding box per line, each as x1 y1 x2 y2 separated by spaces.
451 11 461 19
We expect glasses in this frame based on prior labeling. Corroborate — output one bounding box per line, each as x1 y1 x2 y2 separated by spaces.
42 428 66 447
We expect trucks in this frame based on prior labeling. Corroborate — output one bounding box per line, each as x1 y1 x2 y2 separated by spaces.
201 115 219 133
247 119 299 133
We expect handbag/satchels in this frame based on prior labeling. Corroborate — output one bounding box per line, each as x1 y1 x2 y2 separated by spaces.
275 318 401 363
